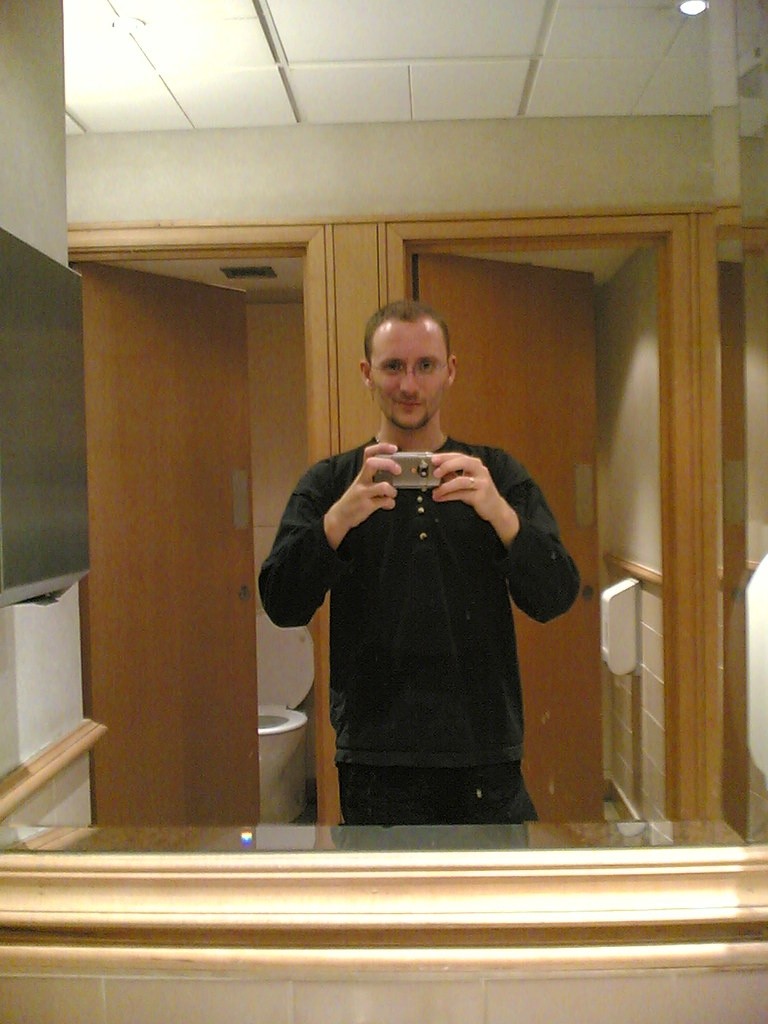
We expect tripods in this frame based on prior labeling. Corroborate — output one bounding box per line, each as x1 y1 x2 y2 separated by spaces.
374 451 442 489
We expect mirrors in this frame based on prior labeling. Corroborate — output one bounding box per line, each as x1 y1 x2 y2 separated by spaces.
0 0 768 853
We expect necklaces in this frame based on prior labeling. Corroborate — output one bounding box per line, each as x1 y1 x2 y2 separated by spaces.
375 436 381 444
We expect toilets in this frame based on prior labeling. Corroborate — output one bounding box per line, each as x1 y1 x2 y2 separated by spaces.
254 602 323 823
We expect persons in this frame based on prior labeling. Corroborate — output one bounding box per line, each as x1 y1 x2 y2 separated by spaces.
258 300 580 823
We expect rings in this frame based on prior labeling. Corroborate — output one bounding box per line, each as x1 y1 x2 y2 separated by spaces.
469 477 474 489
